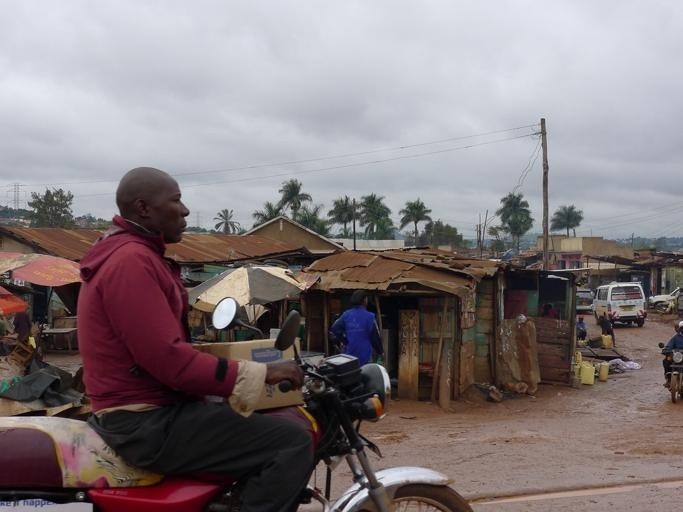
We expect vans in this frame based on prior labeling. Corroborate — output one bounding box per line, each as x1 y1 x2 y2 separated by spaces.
590 279 647 329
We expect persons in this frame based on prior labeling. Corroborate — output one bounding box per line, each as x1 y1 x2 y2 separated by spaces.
544 303 556 318
661 320 683 400
76 164 318 512
576 314 588 340
328 288 386 366
13 311 32 345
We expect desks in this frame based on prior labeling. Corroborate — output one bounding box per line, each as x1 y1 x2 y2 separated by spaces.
41 327 79 355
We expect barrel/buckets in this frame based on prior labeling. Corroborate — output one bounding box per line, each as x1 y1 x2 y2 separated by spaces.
575 350 609 386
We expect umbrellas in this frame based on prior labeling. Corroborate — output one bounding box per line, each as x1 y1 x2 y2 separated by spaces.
187 263 321 325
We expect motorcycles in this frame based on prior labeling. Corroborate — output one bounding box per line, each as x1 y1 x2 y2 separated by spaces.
654 339 682 405
0 294 478 512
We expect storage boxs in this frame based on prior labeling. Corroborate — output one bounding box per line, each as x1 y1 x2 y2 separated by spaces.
192 336 306 411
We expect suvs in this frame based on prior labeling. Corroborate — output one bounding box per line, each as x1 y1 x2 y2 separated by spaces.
575 287 594 315
647 284 681 315
